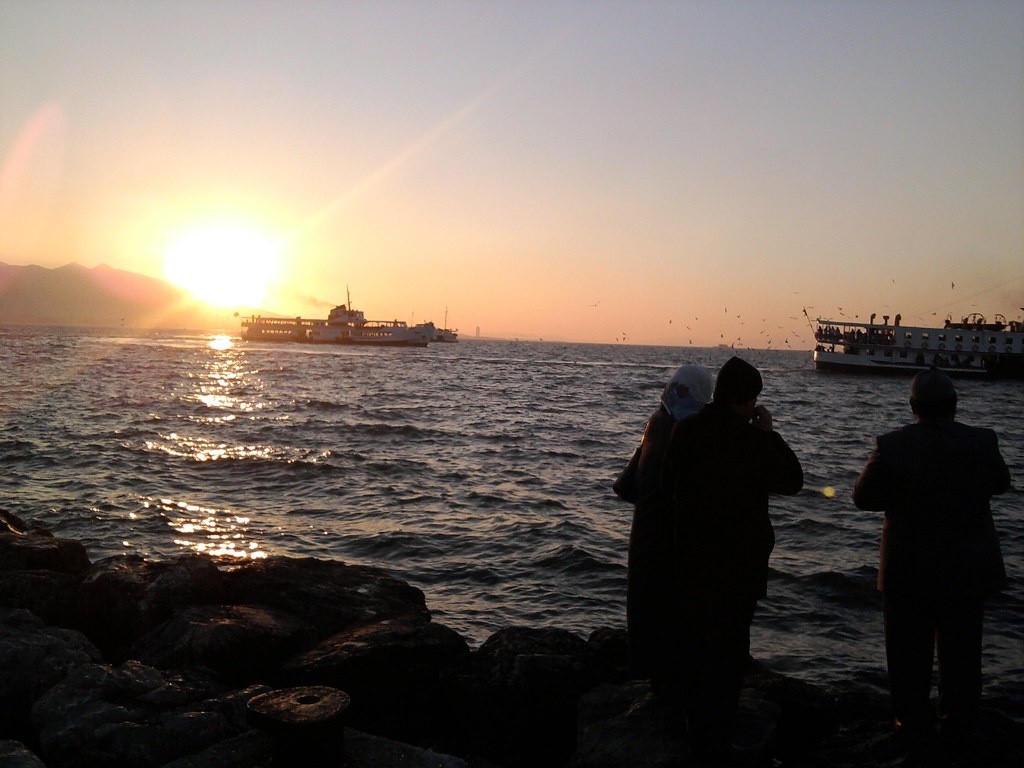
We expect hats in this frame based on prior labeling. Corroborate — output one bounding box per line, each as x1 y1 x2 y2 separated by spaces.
910 365 957 403
660 365 713 420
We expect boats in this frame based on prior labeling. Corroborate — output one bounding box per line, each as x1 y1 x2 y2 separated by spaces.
234 285 434 346
430 306 459 342
802 306 1024 379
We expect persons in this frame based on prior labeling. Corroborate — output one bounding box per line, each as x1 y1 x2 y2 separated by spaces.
852 369 1011 733
816 325 865 344
627 357 804 715
